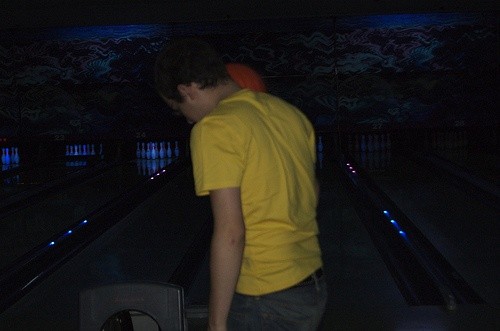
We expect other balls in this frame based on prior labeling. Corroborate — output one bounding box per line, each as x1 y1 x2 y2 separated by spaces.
226 63 266 90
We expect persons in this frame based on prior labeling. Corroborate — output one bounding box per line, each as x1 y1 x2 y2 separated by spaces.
154 39 328 331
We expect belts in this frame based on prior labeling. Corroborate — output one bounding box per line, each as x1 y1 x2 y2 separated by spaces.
301 268 324 283
